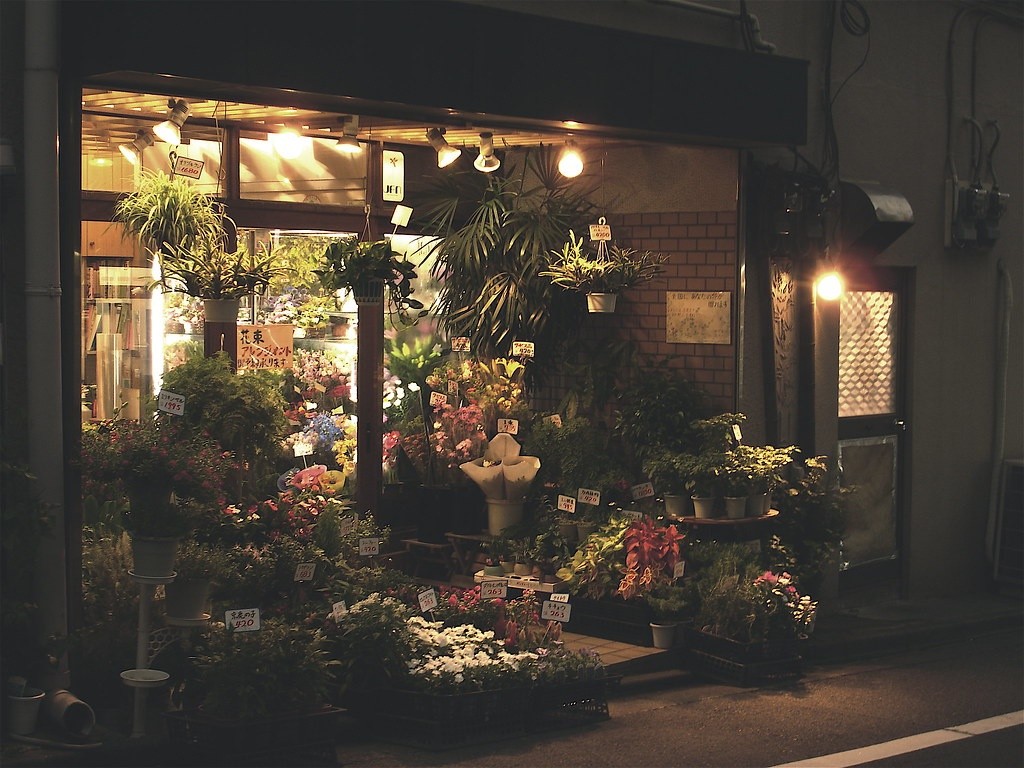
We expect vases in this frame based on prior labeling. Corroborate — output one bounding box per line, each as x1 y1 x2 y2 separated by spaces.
5 686 46 736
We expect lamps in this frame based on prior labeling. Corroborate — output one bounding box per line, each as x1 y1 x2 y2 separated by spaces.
425 128 461 167
118 131 153 166
152 99 190 144
474 131 500 171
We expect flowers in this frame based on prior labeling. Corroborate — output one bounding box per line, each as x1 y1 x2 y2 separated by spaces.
747 536 826 635
80 345 609 688
169 250 351 341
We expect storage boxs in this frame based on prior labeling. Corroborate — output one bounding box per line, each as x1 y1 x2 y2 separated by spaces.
160 738 338 767
684 645 804 687
677 623 810 663
159 704 349 748
367 710 528 751
377 682 541 720
535 672 623 707
527 696 609 732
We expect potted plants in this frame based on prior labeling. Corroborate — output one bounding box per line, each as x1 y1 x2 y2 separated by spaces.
642 413 800 518
544 552 563 581
576 520 598 541
313 235 429 331
141 240 295 323
644 575 687 648
533 533 541 576
514 536 533 574
479 536 504 575
537 228 671 313
558 517 577 540
118 454 197 576
497 528 515 572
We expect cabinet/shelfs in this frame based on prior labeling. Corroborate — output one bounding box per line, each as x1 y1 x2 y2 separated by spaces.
80 255 148 421
